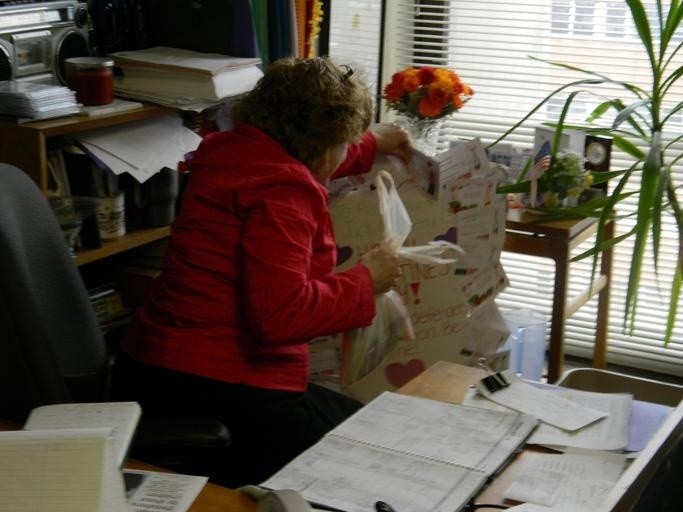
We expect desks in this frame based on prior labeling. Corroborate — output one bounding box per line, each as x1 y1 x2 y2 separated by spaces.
0 422 265 512
494 204 617 384
270 357 683 512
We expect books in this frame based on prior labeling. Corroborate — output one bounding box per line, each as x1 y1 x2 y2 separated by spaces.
0 79 83 124
256 388 545 511
78 97 141 118
108 41 266 118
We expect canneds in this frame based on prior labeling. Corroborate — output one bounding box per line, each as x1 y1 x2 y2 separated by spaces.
66 57 114 105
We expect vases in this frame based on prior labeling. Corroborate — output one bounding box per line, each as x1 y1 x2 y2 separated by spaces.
405 115 450 156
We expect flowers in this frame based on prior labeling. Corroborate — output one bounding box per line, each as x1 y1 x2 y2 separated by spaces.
374 66 475 121
529 150 594 212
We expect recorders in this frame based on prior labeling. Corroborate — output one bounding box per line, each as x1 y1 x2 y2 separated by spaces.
1 1 98 86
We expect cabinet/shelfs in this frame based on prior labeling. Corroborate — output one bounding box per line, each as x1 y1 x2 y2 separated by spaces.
0 103 195 353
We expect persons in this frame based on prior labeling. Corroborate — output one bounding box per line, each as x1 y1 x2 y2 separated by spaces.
120 54 412 490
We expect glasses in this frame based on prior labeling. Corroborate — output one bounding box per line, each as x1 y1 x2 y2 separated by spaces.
337 64 355 83
